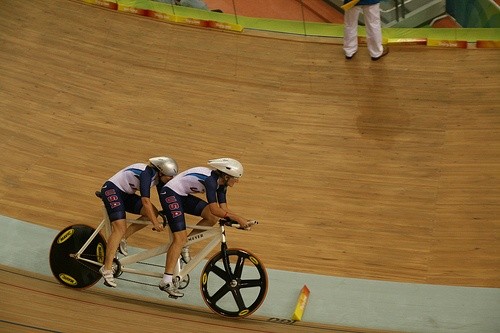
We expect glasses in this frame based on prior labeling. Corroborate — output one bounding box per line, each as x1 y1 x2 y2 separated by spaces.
232 176 238 180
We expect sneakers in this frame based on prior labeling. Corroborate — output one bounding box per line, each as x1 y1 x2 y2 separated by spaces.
158 279 184 297
119 237 128 256
99 265 117 288
180 244 192 265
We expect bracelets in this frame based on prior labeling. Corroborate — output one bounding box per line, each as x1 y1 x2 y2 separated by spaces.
223 211 228 218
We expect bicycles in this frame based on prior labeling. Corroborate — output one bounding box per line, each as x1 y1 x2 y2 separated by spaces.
49 190 269 319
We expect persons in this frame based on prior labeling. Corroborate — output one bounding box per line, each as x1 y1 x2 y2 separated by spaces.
341 0 390 62
98 154 179 289
159 157 254 297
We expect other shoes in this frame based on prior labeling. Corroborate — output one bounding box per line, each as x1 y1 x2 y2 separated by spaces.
346 52 357 59
371 46 390 61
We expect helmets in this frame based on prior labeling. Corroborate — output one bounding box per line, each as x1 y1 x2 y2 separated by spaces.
207 158 244 178
148 156 178 176
